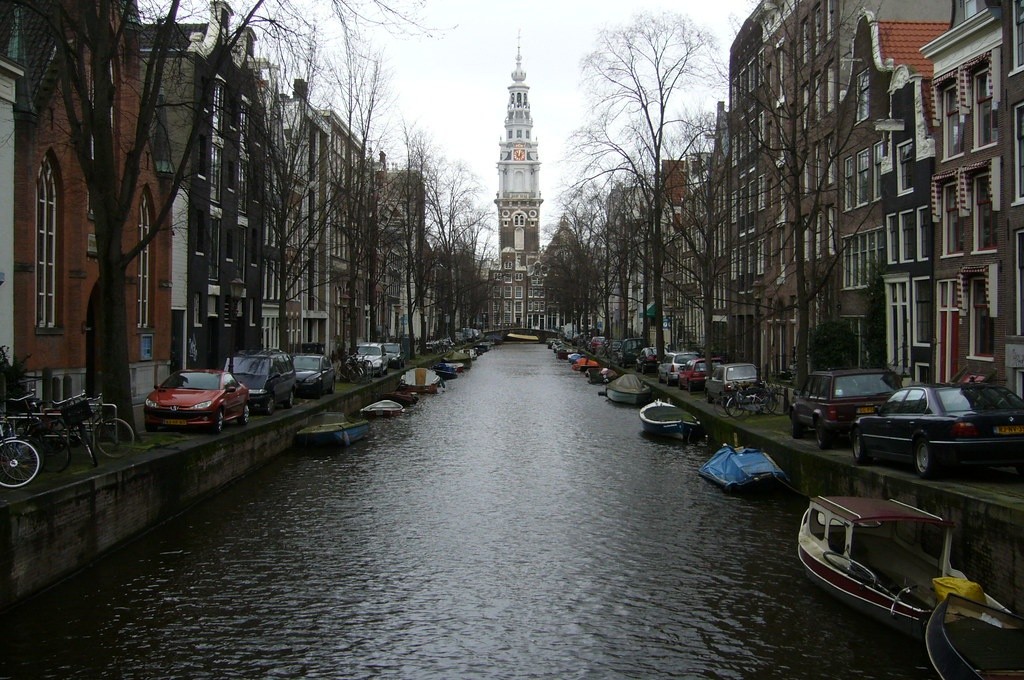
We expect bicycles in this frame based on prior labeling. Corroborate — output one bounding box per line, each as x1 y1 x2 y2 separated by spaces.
714 376 790 419
338 351 374 385
0 388 136 489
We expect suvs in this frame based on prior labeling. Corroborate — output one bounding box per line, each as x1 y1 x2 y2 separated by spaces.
220 347 299 415
788 367 902 450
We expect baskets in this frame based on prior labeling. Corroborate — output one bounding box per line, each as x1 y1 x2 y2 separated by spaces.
59 399 93 426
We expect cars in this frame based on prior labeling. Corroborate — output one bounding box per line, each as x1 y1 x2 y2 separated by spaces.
848 380 1023 478
289 353 336 399
346 341 389 376
383 341 406 370
454 327 481 345
143 367 251 434
564 330 761 402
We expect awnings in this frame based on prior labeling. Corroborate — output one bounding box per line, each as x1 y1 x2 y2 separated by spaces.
639 301 656 318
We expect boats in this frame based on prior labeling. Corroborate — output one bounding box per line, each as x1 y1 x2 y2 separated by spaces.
295 412 370 448
639 398 704 444
546 336 572 359
796 496 1024 680
598 373 653 405
698 432 787 501
584 367 618 384
572 358 599 372
566 353 585 363
360 342 494 418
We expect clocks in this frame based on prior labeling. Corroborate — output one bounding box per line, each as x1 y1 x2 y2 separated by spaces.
514 150 525 161
